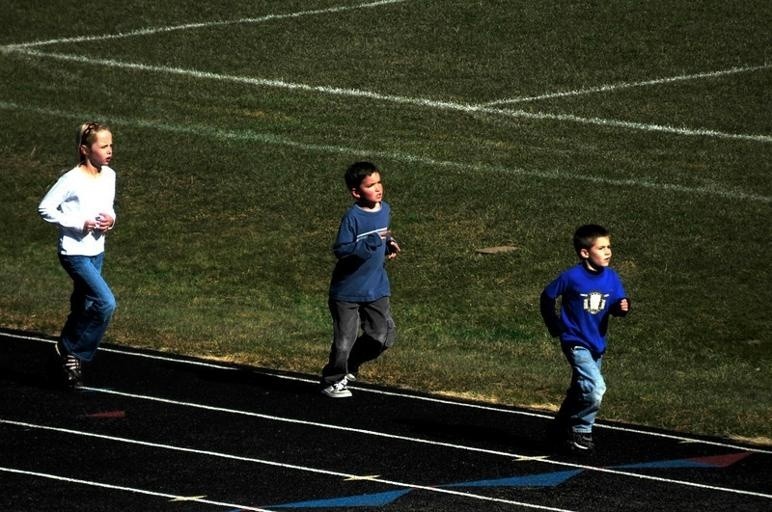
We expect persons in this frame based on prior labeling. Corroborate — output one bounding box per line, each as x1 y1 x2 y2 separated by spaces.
39 121 117 381
320 161 401 398
540 225 632 450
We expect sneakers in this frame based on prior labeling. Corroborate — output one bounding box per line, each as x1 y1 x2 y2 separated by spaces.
569 430 594 450
54 341 82 381
345 373 357 382
321 379 353 399
